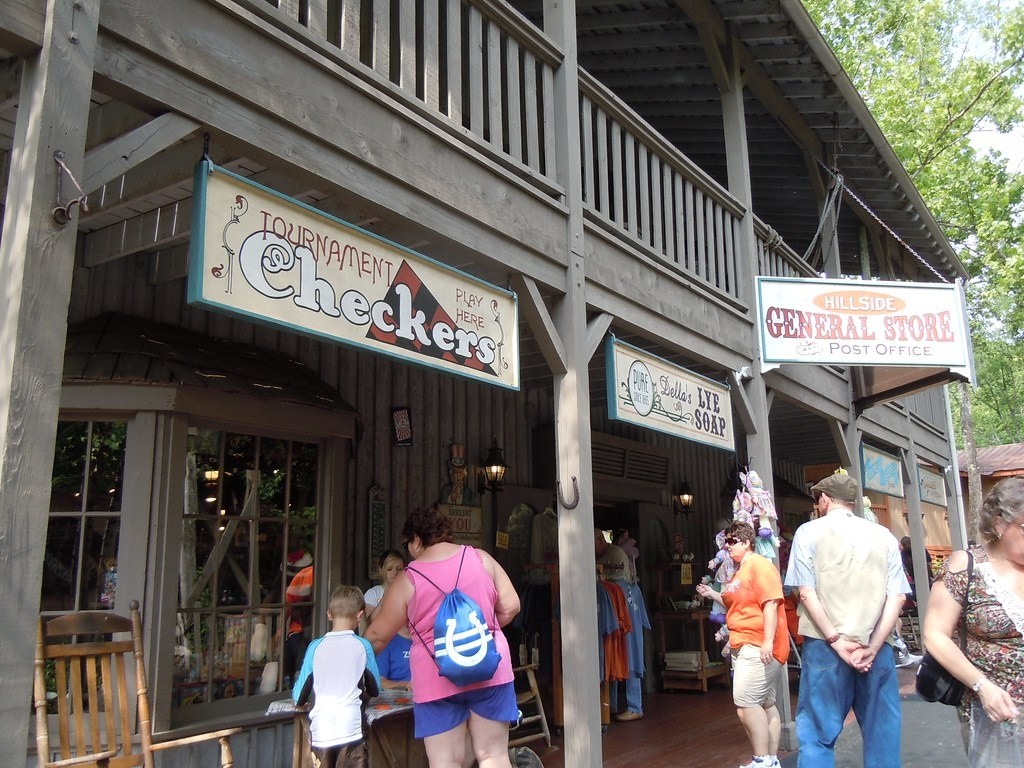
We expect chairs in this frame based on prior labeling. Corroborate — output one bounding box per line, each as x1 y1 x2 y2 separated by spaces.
33 600 244 768
507 664 552 749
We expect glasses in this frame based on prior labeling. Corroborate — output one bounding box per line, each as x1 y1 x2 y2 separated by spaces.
402 540 411 552
724 538 742 547
1012 520 1024 534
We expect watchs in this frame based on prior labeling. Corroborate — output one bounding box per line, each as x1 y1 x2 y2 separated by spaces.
973 679 986 691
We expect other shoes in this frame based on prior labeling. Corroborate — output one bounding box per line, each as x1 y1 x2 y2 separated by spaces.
739 755 773 768
616 710 641 721
770 756 782 768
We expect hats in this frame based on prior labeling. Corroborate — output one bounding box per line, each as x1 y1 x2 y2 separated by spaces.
811 474 858 502
281 548 313 576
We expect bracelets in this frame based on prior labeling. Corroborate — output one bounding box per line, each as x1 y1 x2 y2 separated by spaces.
826 634 840 645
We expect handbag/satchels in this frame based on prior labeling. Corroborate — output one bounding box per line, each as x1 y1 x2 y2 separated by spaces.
916 650 964 706
407 545 502 686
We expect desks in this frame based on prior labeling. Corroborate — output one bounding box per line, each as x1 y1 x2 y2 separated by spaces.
265 687 430 768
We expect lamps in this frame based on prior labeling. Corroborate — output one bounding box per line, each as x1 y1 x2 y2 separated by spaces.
674 480 696 521
476 437 510 495
201 463 219 503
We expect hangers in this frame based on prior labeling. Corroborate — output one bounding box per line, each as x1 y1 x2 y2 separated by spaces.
543 495 556 517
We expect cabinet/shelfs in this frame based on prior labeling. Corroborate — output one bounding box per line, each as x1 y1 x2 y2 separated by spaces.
656 561 729 692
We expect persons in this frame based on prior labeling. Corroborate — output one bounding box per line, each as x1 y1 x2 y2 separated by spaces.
376 625 413 692
695 521 790 768
292 586 380 768
595 529 643 721
785 474 912 768
922 477 1024 768
362 508 520 768
364 550 404 627
901 536 934 579
